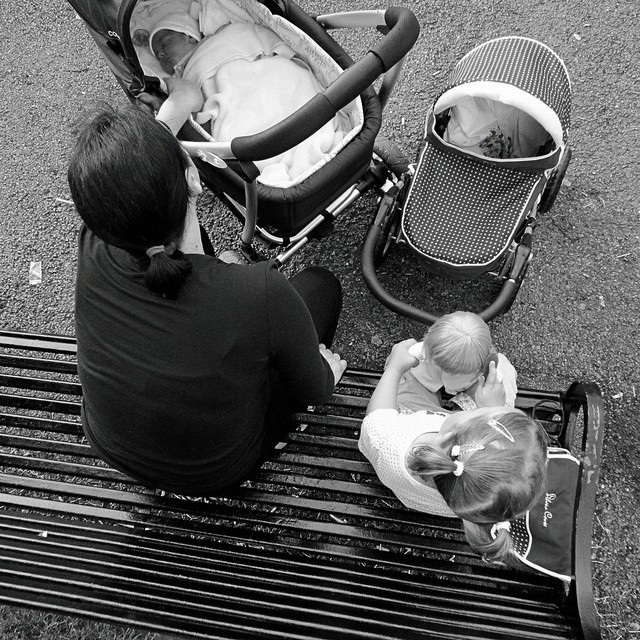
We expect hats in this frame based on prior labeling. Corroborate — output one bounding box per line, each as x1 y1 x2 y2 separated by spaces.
149 11 202 56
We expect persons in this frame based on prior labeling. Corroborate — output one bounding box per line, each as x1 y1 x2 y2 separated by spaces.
358 338 547 564
384 311 518 414
148 13 202 76
67 76 347 497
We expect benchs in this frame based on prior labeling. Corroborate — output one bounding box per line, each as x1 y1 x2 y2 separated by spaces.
1 329 606 639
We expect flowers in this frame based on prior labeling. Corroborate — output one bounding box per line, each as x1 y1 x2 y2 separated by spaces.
479 126 521 159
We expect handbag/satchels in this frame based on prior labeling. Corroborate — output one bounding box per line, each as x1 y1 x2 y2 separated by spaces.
491 446 581 584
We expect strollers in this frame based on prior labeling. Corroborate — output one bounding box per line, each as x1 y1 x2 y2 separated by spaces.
361 37 573 326
59 1 411 268
